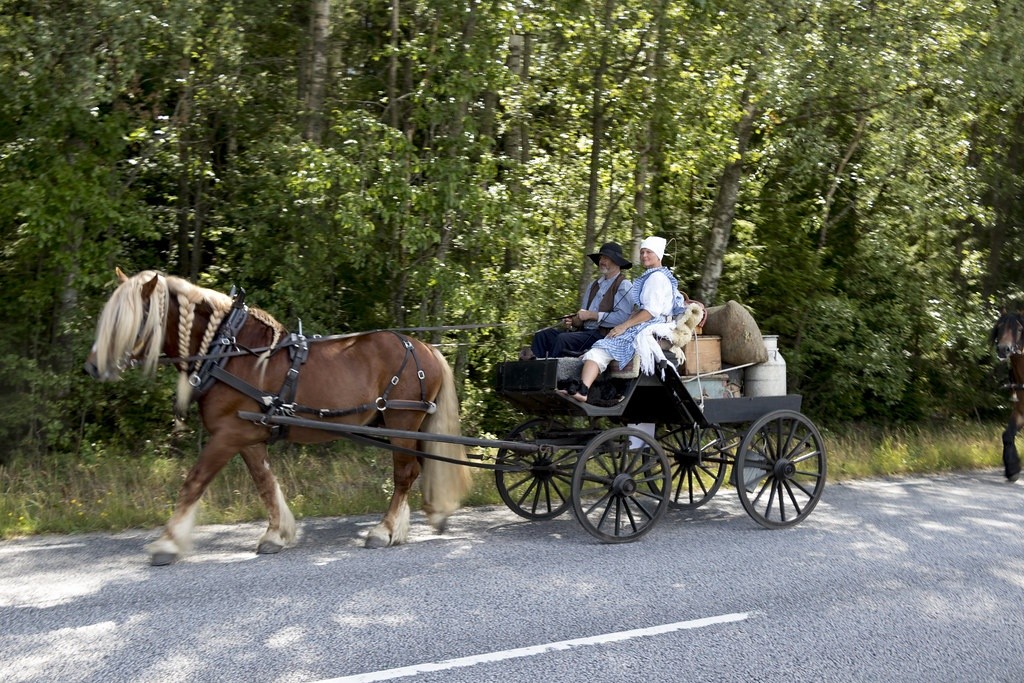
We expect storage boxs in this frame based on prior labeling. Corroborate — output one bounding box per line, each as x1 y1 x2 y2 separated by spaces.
679 374 728 400
683 335 721 374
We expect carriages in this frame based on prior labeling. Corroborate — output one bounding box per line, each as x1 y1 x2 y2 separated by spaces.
78 263 827 568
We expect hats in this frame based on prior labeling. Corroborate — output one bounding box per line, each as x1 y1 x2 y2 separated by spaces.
587 241 632 270
639 236 666 261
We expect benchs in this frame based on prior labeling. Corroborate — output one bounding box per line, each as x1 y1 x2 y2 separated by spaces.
564 291 707 379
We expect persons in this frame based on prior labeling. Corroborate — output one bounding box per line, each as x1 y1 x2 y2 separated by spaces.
558 236 684 404
530 242 634 358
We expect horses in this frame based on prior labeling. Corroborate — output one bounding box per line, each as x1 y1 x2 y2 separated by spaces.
991 307 1024 483
80 264 474 567
